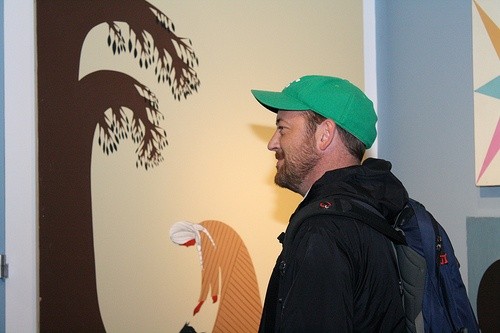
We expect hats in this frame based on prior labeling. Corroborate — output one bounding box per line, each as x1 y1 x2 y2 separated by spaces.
251 75 378 149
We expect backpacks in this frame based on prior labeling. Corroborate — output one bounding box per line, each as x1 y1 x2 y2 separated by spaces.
275 197 480 333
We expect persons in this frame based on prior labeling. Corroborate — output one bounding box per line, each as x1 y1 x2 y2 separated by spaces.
251 75 480 333
